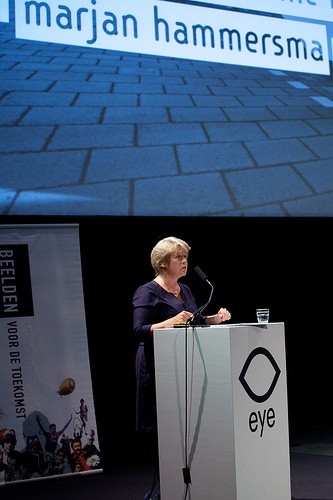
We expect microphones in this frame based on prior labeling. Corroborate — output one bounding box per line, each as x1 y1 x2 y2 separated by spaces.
194 266 212 287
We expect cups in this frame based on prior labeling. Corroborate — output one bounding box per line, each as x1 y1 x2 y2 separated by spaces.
256 308 270 323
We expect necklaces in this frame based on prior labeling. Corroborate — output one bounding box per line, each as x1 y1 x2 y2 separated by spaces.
158 274 179 295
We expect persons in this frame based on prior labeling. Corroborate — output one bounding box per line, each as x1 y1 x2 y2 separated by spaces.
134 236 230 434
0 398 102 486
